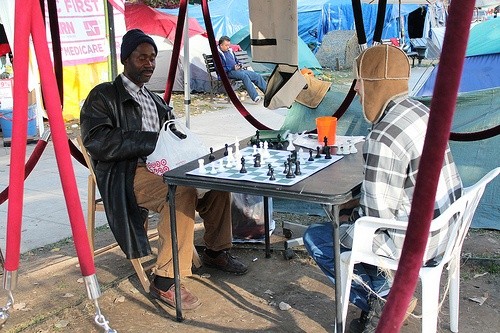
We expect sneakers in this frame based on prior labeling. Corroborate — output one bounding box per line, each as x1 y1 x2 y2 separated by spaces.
350 292 418 333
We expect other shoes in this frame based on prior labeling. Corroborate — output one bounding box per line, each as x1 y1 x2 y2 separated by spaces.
254 97 262 105
150 281 200 310
202 249 248 275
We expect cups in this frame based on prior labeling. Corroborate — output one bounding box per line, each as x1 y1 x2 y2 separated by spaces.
316 117 337 145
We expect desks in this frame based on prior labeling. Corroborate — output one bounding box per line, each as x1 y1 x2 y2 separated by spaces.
162 130 371 333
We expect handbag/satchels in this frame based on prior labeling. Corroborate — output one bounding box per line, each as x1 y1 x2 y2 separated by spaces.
146 120 212 199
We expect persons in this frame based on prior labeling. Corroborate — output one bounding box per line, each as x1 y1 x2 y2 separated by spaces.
80 29 250 311
216 36 267 102
302 43 464 333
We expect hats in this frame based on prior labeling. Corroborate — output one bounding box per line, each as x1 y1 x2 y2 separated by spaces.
121 29 157 64
352 44 410 123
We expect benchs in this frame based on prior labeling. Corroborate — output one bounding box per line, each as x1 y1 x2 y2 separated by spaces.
204 49 251 107
381 39 419 68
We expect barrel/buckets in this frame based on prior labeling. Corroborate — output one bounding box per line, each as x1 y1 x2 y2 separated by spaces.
0 103 37 139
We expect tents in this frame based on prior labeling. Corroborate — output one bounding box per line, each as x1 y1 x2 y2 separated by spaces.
0 0 500 230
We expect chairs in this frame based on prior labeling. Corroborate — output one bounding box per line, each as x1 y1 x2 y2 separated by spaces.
337 165 500 333
77 135 204 293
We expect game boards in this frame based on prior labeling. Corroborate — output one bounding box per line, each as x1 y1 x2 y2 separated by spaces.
185 147 344 186
